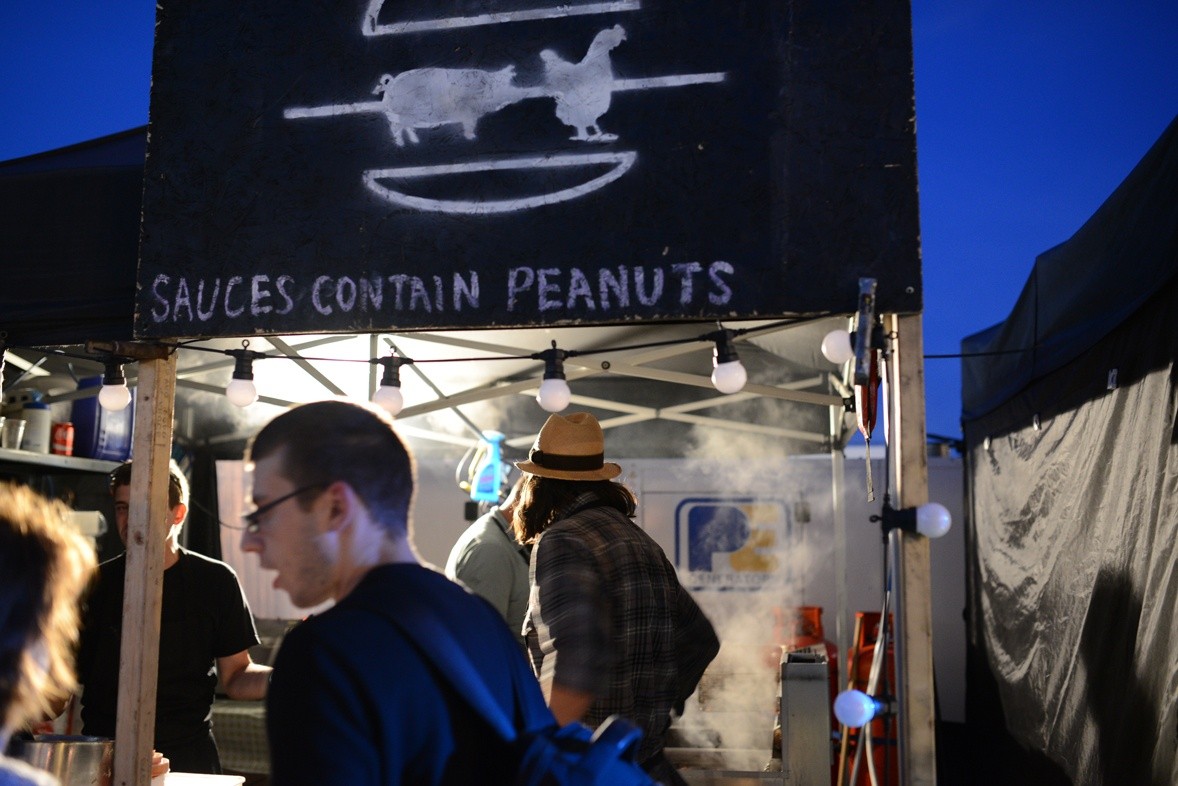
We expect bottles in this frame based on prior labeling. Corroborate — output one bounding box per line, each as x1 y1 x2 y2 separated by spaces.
21 402 51 453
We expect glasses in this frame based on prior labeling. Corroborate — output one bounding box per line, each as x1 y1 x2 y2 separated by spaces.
242 475 349 536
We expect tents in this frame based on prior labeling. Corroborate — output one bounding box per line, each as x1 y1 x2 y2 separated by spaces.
959 112 1178 786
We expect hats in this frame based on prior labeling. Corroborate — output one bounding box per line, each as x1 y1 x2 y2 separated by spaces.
514 411 623 481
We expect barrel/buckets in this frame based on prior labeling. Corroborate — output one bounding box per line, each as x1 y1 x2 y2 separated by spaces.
26 740 115 786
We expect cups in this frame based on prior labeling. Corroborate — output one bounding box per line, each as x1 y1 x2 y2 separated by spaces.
2 419 26 450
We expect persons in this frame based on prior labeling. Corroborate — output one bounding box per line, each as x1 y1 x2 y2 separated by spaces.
38 457 273 775
513 413 720 786
447 472 533 660
0 483 98 786
239 400 538 786
